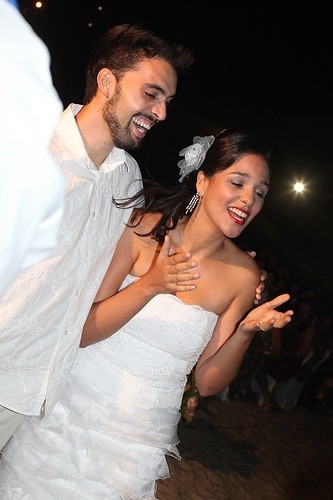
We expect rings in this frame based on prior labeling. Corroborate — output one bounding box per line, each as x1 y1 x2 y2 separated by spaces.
260 325 269 332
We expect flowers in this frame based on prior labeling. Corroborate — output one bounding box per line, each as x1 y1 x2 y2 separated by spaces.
178 134 214 181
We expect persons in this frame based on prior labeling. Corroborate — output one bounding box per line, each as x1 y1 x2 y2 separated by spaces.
0 0 294 500
200 250 333 408
